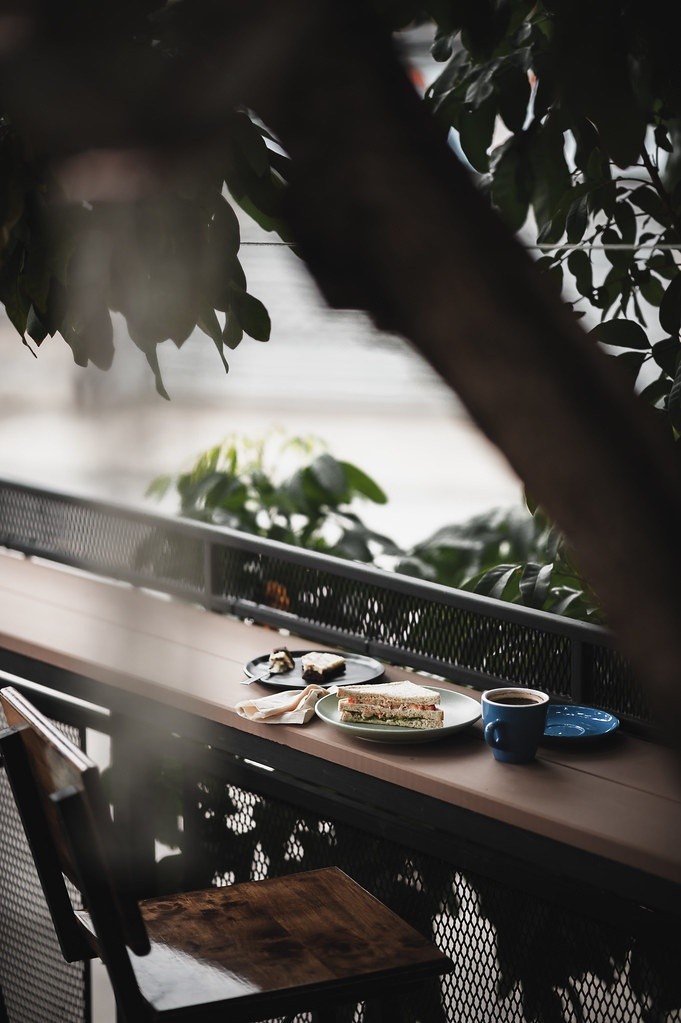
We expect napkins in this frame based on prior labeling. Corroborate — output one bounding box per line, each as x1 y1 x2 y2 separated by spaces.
234 683 340 726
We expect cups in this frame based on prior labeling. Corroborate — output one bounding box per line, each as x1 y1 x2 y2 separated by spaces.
481 687 550 762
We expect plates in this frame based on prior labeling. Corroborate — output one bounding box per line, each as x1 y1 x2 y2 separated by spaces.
542 705 619 743
315 685 482 743
244 651 386 689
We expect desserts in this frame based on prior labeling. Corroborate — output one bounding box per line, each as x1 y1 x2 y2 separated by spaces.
300 652 346 684
270 648 295 672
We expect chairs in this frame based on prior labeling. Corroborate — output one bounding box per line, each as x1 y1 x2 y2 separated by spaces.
0 686 456 1023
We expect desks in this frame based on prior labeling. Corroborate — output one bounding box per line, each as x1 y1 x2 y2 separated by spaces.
0 553 681 953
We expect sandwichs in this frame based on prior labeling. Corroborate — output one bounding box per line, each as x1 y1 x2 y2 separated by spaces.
336 680 444 730
